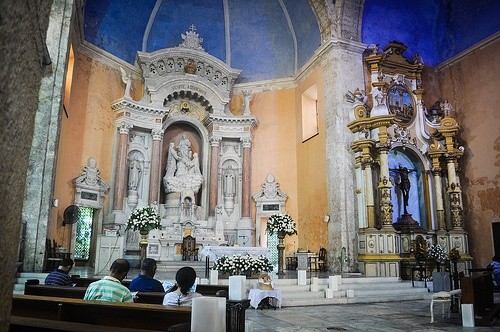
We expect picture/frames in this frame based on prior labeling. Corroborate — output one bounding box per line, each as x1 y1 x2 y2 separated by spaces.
387 80 418 128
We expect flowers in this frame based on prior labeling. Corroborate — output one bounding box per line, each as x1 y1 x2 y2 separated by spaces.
429 243 444 261
214 252 274 277
263 214 298 236
127 206 162 230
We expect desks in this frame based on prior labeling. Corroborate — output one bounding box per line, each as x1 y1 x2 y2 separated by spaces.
411 264 443 289
249 288 282 310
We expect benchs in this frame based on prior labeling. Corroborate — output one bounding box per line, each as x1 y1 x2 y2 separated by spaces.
10 273 251 332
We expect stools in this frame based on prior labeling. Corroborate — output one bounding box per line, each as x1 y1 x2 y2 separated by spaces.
429 291 452 323
449 289 463 313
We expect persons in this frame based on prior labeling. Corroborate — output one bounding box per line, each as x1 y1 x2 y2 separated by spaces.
45 257 74 286
390 167 418 206
82 258 133 303
162 267 203 306
164 134 201 177
128 258 165 292
490 255 500 286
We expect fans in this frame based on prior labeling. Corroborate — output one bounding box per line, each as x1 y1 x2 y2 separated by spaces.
62 204 81 227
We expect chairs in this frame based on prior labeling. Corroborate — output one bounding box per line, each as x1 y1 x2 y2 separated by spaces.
309 247 328 272
286 248 299 270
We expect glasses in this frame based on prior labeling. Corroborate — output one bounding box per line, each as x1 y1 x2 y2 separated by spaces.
124 274 128 279
